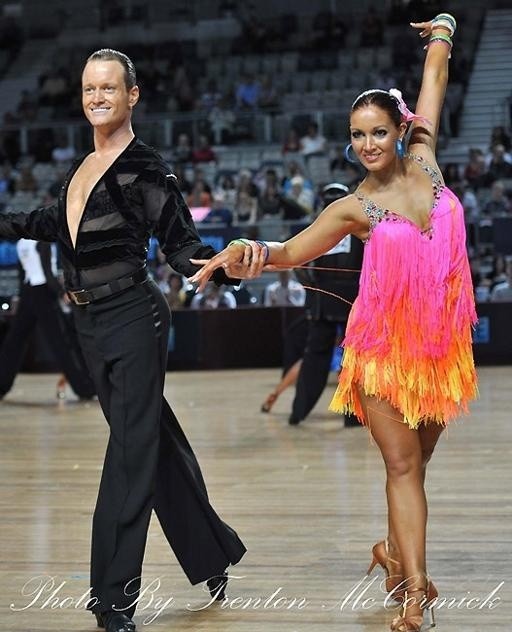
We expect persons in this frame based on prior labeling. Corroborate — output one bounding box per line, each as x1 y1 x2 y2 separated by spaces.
186 12 482 631
1 47 276 631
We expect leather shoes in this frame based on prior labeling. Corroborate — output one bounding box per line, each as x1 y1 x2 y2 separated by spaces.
96 607 137 632
260 393 365 428
206 529 247 602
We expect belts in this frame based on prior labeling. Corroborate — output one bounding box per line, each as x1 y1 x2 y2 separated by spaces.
68 268 150 304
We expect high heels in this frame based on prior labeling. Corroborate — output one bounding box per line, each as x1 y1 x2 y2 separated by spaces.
389 579 440 630
365 538 407 603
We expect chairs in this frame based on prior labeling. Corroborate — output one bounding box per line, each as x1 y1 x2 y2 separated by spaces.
0 1 512 314
